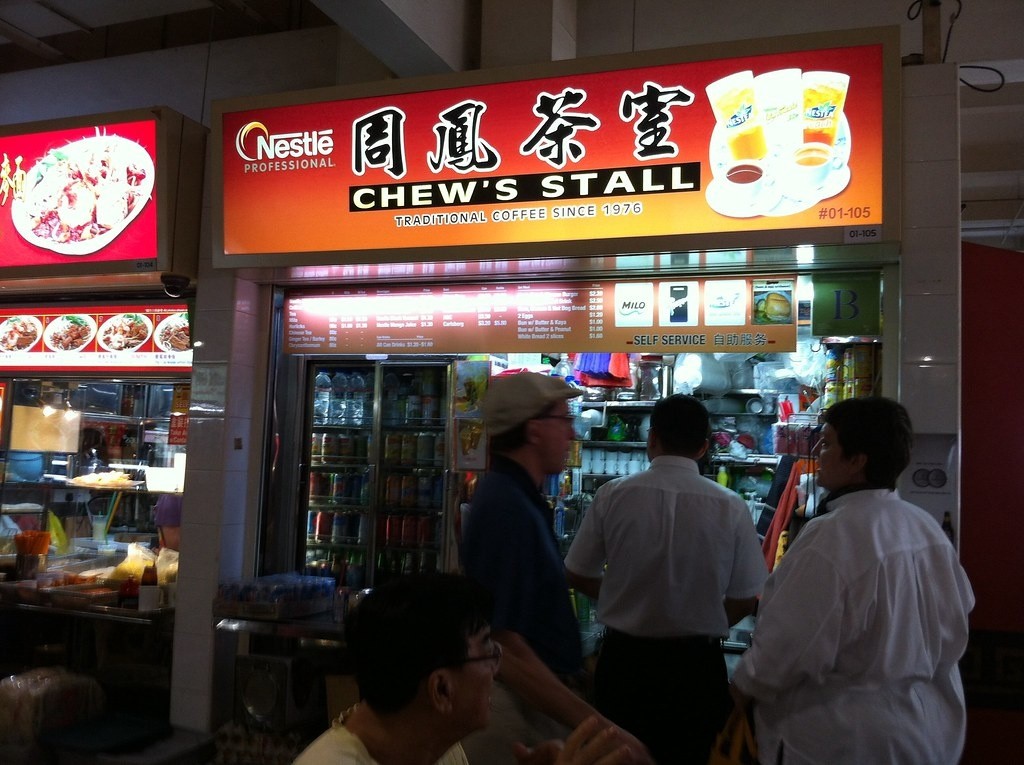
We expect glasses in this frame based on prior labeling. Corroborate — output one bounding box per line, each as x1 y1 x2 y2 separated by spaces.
435 639 503 674
532 413 576 423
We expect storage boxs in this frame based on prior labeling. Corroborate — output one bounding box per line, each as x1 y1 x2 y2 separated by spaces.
0 533 150 610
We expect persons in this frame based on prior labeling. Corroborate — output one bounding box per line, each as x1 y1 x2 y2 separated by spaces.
456 367 977 765
62 428 121 541
292 570 654 765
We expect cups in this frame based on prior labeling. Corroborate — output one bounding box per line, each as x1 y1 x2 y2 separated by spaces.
580 449 652 476
801 72 850 165
708 70 764 161
753 68 801 164
795 141 844 188
727 160 768 205
138 586 163 610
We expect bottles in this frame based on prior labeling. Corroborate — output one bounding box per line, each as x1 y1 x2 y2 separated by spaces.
313 369 446 427
717 467 728 488
639 356 662 400
578 594 590 621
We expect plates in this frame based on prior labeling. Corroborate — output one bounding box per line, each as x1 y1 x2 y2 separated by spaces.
0 317 43 353
787 164 852 202
96 314 152 353
12 138 155 255
706 176 784 217
44 314 96 352
153 314 192 351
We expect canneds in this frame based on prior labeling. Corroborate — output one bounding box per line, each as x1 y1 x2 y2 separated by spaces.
773 345 872 455
305 396 446 585
221 575 374 624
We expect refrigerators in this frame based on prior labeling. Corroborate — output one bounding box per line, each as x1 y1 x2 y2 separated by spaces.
290 351 456 589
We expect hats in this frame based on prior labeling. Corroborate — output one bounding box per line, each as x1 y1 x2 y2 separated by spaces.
480 372 584 437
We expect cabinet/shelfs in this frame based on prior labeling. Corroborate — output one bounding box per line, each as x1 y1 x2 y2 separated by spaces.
0 381 188 534
290 347 846 585
